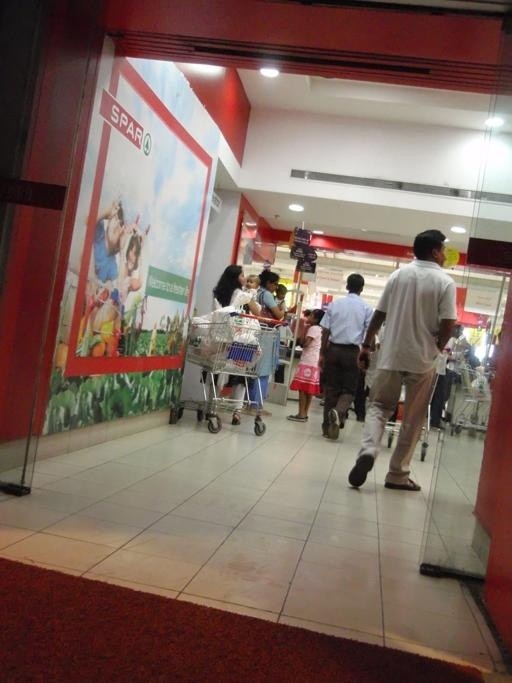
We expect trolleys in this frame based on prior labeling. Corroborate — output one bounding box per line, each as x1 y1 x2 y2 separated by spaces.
384 349 449 462
449 365 490 440
177 314 288 435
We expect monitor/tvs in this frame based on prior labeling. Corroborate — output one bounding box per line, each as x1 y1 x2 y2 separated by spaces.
466 237 512 269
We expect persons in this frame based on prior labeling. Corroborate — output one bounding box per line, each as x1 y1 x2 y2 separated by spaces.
426 324 502 433
318 273 375 439
101 221 150 304
206 264 287 425
82 192 129 306
287 309 399 429
347 228 457 492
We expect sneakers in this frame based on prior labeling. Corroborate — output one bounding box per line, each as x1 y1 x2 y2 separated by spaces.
323 407 340 440
287 414 309 422
245 406 271 416
349 453 374 487
385 477 421 491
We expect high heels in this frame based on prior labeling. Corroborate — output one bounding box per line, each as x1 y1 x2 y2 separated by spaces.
232 412 240 425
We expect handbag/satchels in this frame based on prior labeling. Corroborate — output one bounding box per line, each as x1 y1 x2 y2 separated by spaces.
260 307 276 326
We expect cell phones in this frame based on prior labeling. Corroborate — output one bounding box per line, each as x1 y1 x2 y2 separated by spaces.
359 352 370 369
